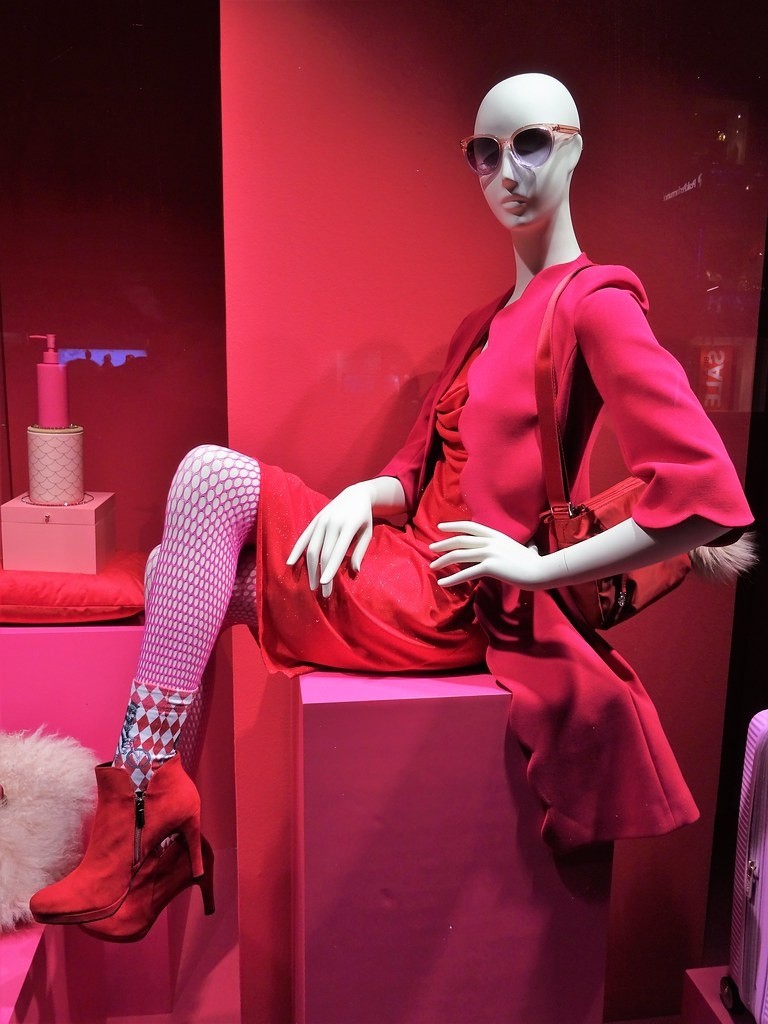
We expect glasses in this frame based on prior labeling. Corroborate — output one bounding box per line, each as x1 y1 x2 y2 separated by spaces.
459 122 581 175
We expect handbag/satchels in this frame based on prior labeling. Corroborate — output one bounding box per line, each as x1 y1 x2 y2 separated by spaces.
534 263 693 629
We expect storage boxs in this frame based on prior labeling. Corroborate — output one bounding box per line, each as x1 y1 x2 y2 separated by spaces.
1 491 114 574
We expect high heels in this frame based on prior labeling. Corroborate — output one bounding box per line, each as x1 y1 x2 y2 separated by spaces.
29 751 214 943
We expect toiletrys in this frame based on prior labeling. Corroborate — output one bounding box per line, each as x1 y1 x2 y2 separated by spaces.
29 332 68 429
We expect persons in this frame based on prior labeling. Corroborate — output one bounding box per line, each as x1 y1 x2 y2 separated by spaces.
28 73 756 943
